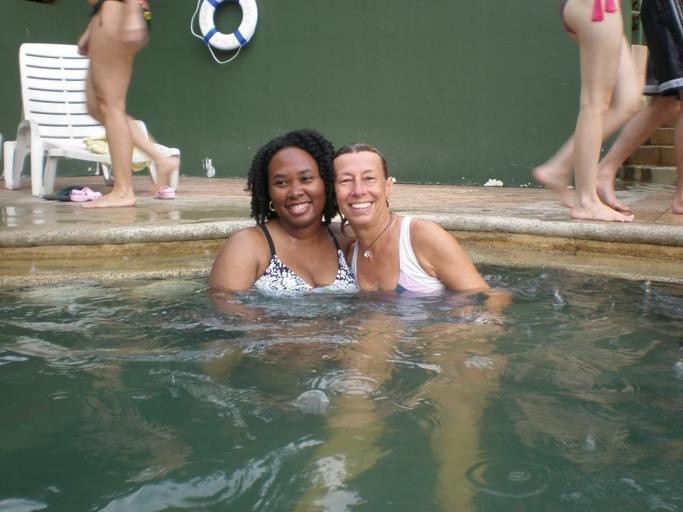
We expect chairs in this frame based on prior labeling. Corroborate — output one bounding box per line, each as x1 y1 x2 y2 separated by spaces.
3 43 180 198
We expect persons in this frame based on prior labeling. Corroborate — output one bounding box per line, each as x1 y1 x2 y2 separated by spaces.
296 141 514 512
76 0 180 209
596 0 683 215
210 128 361 319
529 0 645 223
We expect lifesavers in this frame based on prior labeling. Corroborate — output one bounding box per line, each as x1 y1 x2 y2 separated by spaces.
198 0 258 51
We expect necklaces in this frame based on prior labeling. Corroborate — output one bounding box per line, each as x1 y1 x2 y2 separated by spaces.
359 215 395 258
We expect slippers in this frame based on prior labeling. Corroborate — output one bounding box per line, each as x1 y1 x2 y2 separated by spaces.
42 185 103 201
155 185 175 199
